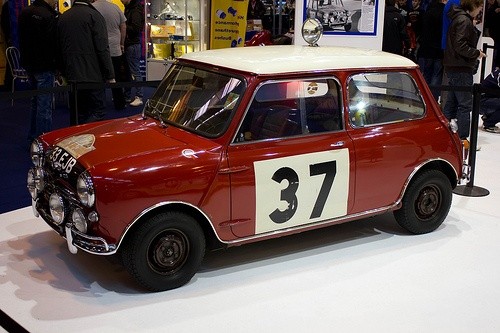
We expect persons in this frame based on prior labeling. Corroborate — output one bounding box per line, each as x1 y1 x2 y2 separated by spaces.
479 67 500 133
444 0 488 152
91 0 128 112
17 1 60 140
248 0 295 33
481 0 500 82
112 0 144 106
382 0 466 114
59 1 116 127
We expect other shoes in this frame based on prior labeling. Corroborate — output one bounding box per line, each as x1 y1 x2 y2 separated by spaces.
462 139 481 150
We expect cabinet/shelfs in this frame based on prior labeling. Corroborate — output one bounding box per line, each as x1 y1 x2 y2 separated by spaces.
145 0 204 81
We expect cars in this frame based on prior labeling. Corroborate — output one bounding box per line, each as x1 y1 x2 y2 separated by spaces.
29 18 465 290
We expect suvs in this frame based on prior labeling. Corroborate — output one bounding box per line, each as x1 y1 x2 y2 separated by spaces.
307 0 352 31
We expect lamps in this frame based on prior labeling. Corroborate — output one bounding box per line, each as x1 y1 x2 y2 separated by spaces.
302 17 323 47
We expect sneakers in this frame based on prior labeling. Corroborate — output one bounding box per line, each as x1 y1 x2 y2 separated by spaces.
130 97 143 106
482 124 500 133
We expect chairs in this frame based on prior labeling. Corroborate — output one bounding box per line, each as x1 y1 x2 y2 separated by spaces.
6 46 29 92
254 105 291 139
